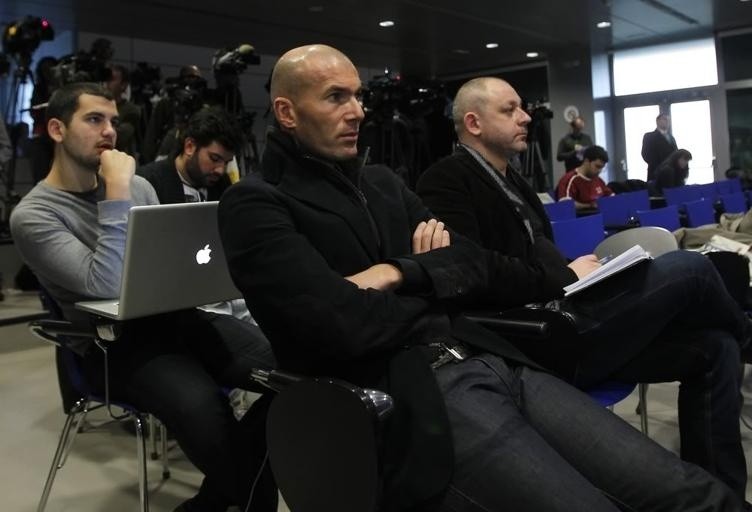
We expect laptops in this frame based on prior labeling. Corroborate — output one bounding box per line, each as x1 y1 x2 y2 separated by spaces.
74 201 244 321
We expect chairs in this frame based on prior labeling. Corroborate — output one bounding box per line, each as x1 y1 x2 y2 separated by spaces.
18 313 171 511
247 364 395 509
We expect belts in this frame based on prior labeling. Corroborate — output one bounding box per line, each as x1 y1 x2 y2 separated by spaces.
432 338 478 368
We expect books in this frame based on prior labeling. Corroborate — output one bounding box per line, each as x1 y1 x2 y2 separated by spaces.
561 245 655 298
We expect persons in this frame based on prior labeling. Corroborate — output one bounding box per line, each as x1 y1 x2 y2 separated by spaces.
10 84 279 511
416 78 752 499
217 43 752 511
0 39 752 349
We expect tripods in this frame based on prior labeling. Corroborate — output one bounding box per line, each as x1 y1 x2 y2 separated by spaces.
234 131 264 177
525 141 551 192
4 62 36 233
